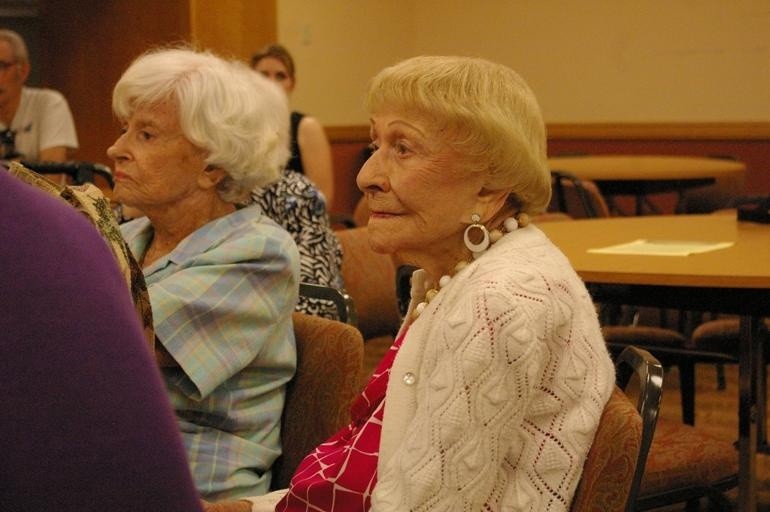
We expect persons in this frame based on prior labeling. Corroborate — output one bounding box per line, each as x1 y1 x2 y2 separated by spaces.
103 27 303 502
240 165 351 326
0 30 80 184
250 42 333 214
199 54 616 512
0 163 202 511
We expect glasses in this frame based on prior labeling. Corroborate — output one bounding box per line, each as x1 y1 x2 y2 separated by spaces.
0 60 16 71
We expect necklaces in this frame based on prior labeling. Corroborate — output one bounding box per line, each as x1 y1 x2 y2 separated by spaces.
408 212 529 320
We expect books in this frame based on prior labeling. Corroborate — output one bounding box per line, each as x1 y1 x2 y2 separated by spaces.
585 236 735 260
